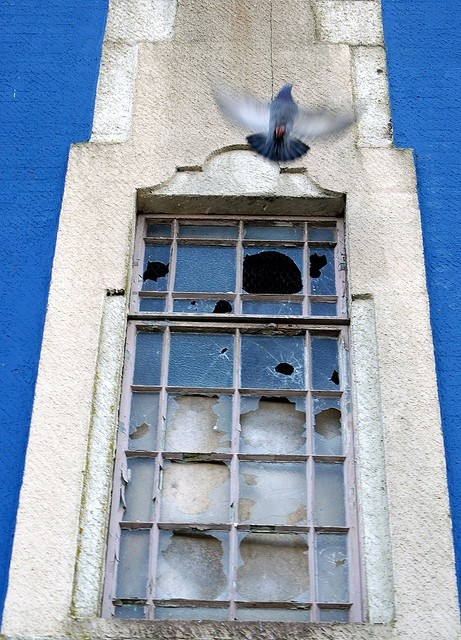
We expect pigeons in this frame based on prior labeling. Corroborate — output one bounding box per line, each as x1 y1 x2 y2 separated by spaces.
205 77 364 165
